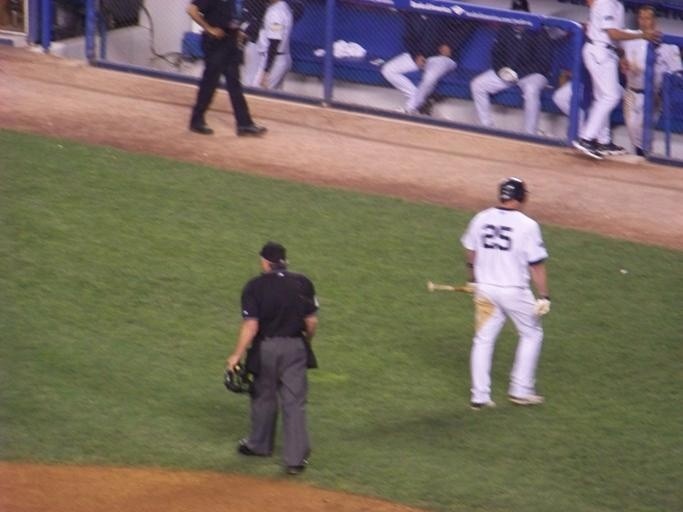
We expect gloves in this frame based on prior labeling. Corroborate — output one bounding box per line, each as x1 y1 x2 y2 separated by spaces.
534 296 551 316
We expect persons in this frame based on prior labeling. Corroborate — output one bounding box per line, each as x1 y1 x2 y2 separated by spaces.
469 0 554 135
379 11 478 118
186 1 267 137
225 240 320 475
460 176 552 412
252 0 294 92
551 1 683 162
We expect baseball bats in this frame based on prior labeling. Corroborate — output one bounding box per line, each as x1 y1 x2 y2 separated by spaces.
428 281 475 293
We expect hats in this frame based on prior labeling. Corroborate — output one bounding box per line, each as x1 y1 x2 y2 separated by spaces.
259 241 286 263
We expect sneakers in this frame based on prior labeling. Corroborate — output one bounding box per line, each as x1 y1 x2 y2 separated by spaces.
471 400 496 411
237 122 266 136
572 137 627 160
507 392 544 405
190 114 214 134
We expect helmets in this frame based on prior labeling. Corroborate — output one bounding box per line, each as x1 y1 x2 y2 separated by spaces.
498 177 528 202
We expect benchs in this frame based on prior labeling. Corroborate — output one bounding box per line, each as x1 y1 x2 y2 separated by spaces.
181 0 682 156
